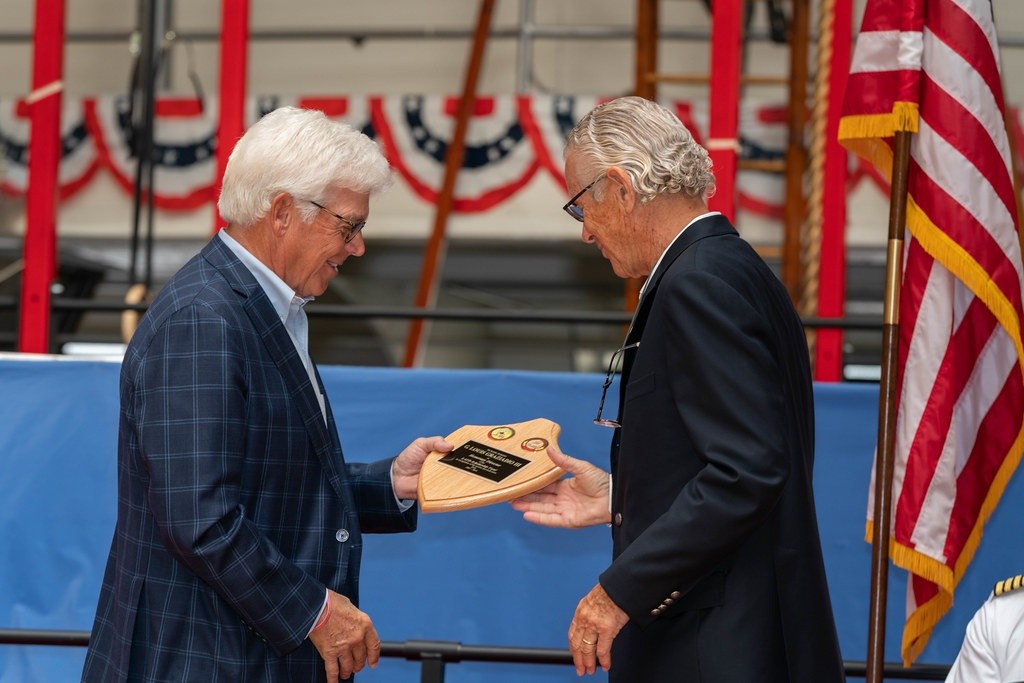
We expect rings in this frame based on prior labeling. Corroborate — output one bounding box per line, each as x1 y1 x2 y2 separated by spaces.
582 639 596 645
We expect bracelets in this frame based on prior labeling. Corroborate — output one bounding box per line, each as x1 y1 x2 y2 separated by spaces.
313 598 331 633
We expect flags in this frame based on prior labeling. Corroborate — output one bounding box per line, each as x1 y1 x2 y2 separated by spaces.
840 0 1024 667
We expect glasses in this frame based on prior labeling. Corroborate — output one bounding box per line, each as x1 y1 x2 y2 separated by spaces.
562 176 604 223
311 201 367 244
594 341 640 428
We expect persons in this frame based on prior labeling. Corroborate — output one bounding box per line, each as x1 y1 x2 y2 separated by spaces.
512 96 846 683
944 574 1024 683
81 106 452 683
